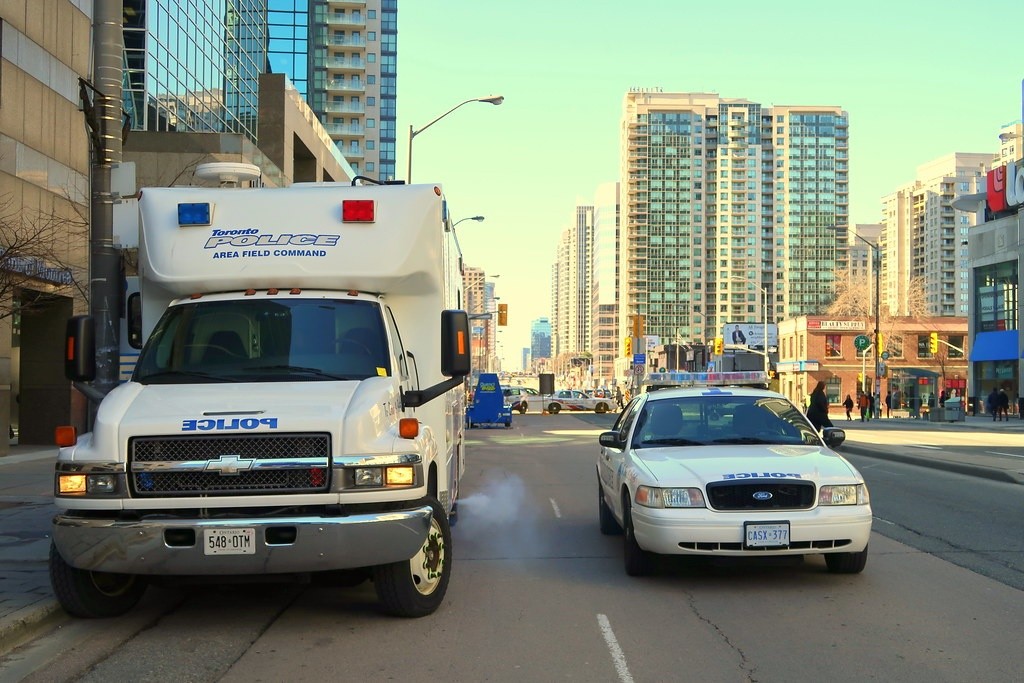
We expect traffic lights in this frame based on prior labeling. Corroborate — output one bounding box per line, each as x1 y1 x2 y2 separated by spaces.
930 332 938 353
624 336 632 358
713 338 723 355
769 371 776 377
858 372 864 382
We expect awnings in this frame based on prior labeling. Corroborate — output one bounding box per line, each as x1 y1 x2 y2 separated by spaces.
969 330 1019 361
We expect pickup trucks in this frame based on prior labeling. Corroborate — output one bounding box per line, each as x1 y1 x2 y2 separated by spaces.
527 389 617 414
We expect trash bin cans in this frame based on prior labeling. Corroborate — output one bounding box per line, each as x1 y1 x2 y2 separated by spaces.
943 397 965 421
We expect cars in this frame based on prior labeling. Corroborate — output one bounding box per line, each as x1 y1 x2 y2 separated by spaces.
592 372 874 577
496 387 541 415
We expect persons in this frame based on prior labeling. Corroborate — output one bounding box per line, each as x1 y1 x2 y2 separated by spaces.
858 391 875 421
732 325 746 344
843 395 853 420
616 387 624 409
885 391 891 418
939 391 946 407
928 392 935 407
988 388 998 421
998 389 1009 421
806 381 833 432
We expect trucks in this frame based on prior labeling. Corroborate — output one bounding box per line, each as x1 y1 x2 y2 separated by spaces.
47 178 471 619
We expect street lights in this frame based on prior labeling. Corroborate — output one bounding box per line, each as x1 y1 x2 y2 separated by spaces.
407 96 504 188
846 225 881 420
731 275 770 389
